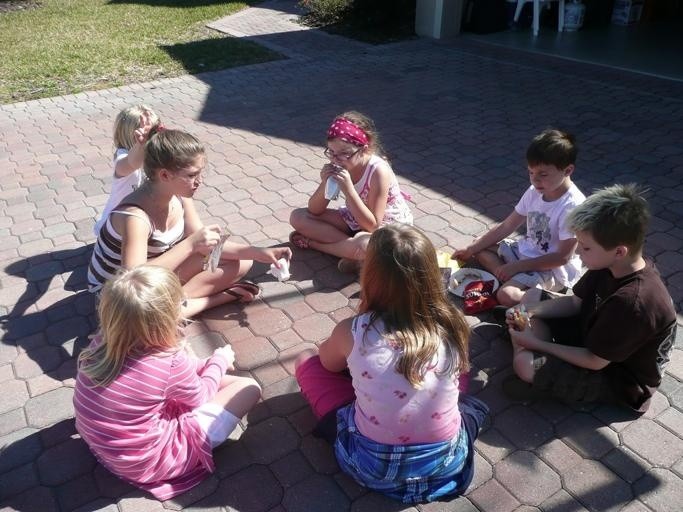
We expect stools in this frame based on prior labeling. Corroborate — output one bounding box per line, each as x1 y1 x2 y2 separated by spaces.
513 0 565 36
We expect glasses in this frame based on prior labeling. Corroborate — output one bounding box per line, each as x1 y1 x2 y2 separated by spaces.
324 148 362 161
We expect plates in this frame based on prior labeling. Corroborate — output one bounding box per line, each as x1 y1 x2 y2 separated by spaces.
447 267 500 299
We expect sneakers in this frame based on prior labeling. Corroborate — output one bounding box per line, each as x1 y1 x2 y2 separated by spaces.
290 232 311 248
339 258 354 272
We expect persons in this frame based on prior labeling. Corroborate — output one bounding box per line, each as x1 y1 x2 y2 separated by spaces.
290 111 414 276
87 124 293 321
451 129 588 305
72 264 262 501
294 223 489 504
93 105 161 238
506 184 677 413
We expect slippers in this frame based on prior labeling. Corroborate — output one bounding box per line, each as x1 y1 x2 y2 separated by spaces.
224 281 259 303
